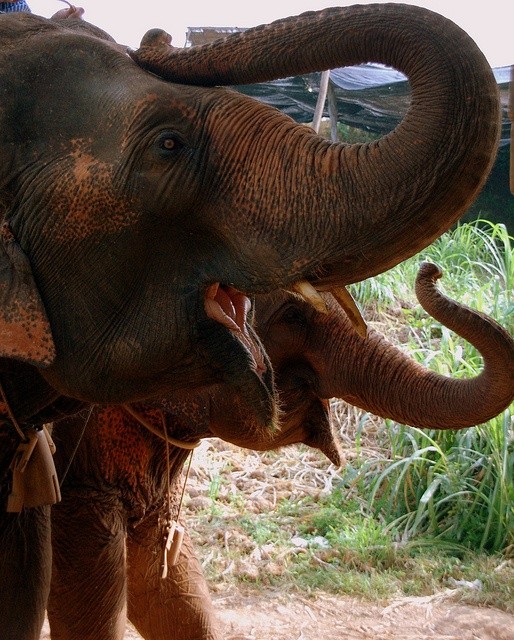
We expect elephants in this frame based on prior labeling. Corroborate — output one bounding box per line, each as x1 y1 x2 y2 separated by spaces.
0 4 502 640
45 264 514 640
